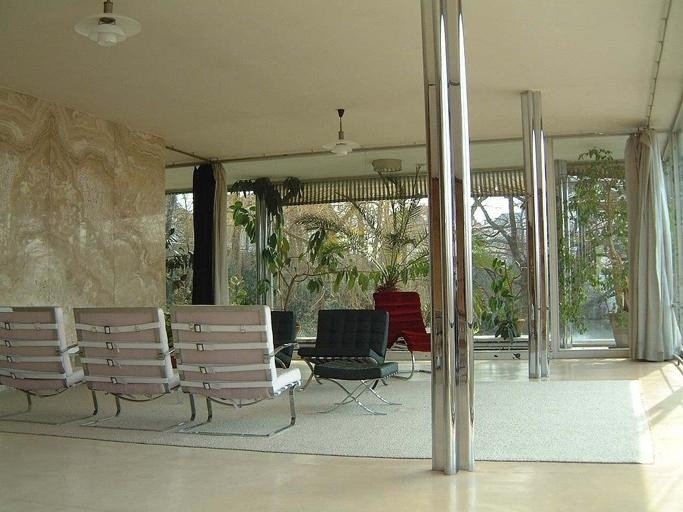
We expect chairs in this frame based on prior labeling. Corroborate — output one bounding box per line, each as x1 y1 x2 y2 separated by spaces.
0 303 100 427
169 302 303 440
270 309 299 367
71 305 199 433
298 306 390 395
372 291 432 382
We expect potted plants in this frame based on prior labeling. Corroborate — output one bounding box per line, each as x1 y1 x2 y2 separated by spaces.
481 258 525 343
228 177 379 339
557 148 633 347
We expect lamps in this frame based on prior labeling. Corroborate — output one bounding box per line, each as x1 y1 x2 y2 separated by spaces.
74 0 141 46
372 159 402 172
323 109 360 156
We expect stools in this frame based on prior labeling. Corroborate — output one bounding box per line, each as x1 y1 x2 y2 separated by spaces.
313 360 405 417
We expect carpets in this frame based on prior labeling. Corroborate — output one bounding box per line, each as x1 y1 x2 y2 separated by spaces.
0 365 654 465
